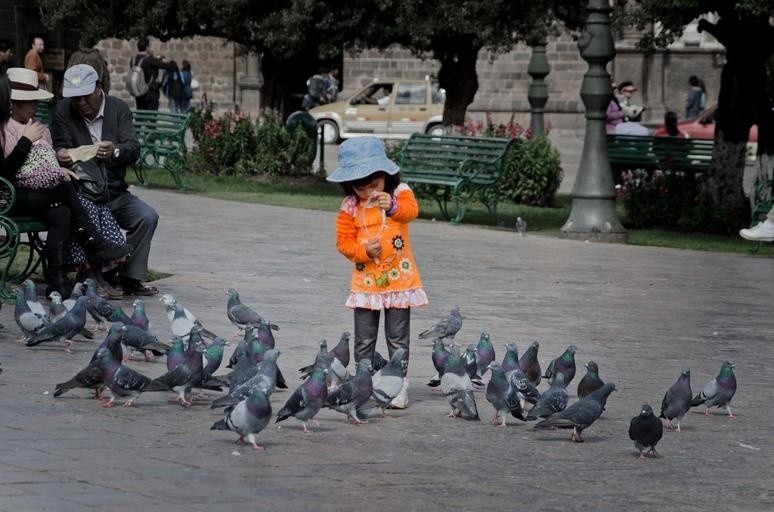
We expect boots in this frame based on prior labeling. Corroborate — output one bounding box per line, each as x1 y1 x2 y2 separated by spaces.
70 206 134 272
45 240 88 302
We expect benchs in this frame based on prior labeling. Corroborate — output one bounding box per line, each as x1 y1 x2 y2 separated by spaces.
0 174 55 304
33 97 52 130
127 109 193 189
745 177 774 256
391 131 516 224
607 133 714 182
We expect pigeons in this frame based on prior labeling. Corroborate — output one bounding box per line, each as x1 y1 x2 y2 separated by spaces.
657 369 692 432
11 278 289 412
690 360 738 419
516 215 528 239
417 331 618 446
628 404 664 459
418 305 463 342
274 362 330 433
297 330 406 423
211 382 273 452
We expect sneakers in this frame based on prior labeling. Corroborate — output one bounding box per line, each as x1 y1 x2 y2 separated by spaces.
389 387 409 409
738 221 774 242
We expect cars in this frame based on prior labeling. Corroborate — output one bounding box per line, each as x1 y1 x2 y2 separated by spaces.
655 100 759 144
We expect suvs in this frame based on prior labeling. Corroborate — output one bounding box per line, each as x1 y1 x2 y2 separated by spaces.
306 74 446 146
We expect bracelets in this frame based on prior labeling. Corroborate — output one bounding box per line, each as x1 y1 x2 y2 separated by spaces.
386 200 398 216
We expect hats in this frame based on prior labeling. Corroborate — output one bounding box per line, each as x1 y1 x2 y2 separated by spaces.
6 68 55 101
620 85 638 92
626 104 645 120
325 136 400 183
62 64 99 98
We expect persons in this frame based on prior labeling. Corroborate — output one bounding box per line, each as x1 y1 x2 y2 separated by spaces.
362 89 391 105
609 76 707 180
302 67 328 111
327 66 339 103
326 136 429 408
739 199 774 242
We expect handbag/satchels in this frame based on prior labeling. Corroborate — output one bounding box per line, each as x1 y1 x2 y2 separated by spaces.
166 78 191 100
65 157 110 204
301 94 313 111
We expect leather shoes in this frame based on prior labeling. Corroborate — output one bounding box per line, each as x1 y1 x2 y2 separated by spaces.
94 279 160 301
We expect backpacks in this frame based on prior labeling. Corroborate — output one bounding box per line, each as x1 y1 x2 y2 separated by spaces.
126 55 155 97
